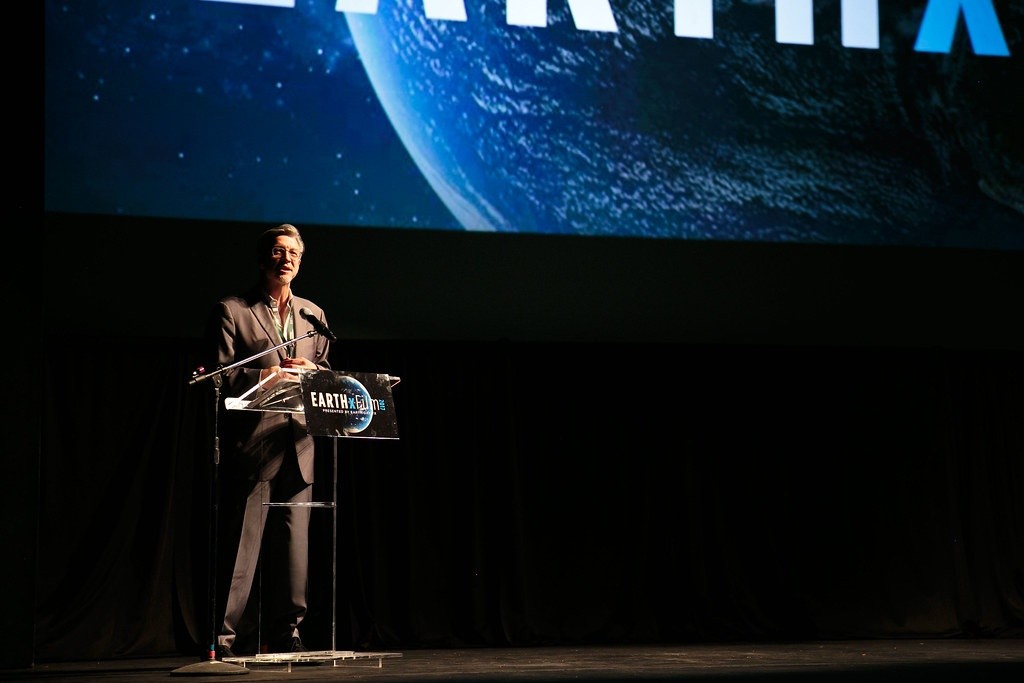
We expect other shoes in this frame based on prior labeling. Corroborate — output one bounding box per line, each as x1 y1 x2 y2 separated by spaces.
267 638 308 653
200 644 244 667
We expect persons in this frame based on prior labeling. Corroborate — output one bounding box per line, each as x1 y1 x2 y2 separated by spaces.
213 224 332 657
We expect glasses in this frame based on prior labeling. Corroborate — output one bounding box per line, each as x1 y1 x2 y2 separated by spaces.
261 246 301 258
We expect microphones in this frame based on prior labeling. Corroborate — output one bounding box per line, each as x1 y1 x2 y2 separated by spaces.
299 307 337 342
